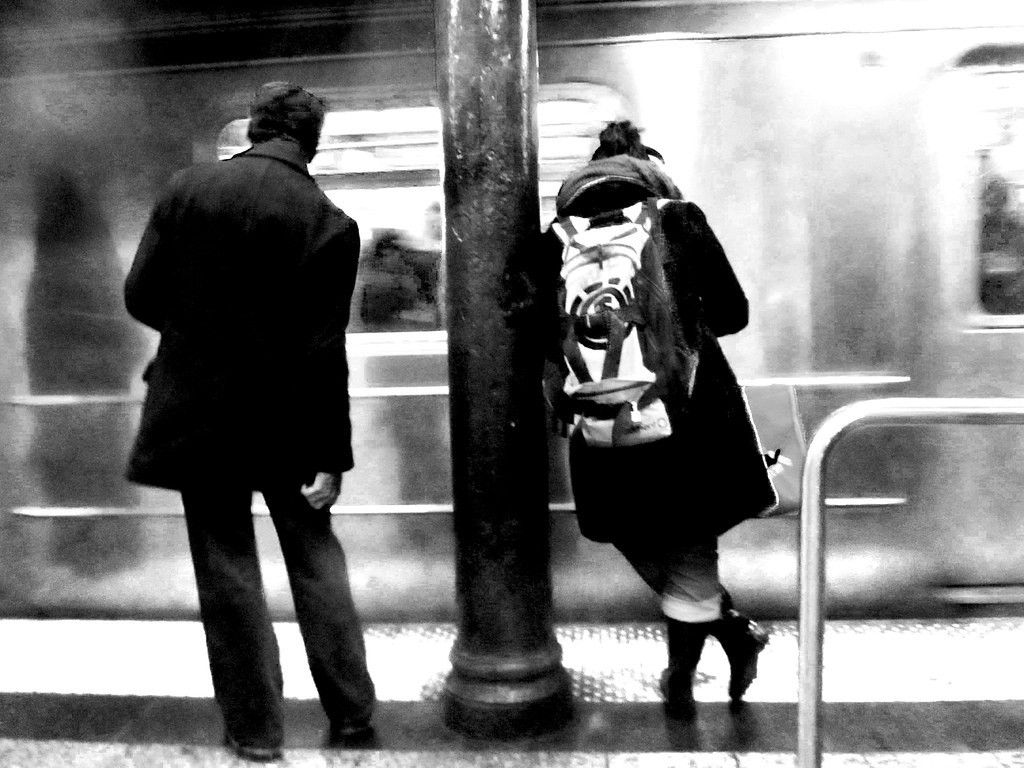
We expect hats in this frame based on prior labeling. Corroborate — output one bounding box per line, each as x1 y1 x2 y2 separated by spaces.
248 82 325 124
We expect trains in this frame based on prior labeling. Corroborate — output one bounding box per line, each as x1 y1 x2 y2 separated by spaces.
1 0 1024 619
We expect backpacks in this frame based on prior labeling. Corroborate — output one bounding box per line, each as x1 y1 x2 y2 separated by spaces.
542 198 702 446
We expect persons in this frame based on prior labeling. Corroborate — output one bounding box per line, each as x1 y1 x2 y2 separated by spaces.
973 169 1024 315
362 226 422 327
536 117 780 722
121 78 379 759
17 160 146 578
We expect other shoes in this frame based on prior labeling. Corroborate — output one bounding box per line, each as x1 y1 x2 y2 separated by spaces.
727 620 769 703
219 717 378 758
659 668 699 721
